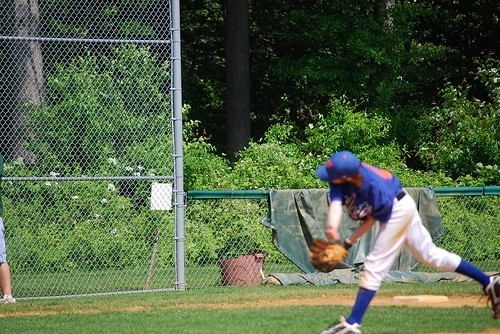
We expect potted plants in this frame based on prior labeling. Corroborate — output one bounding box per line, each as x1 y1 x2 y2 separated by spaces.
214 231 269 287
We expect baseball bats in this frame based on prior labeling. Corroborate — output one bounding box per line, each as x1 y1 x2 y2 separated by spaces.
144 232 160 290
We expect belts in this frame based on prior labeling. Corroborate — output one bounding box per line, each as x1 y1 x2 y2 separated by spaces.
396 192 405 201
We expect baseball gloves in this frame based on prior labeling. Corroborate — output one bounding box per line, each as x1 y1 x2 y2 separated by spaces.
309 238 350 274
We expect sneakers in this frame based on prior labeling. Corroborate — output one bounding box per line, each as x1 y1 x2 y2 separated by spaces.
319 316 363 334
0 295 16 304
477 276 500 319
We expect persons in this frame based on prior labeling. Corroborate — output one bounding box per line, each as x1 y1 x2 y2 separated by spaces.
0 154 16 305
316 150 500 334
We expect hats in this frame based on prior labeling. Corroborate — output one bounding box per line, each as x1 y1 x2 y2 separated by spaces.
316 151 359 181
